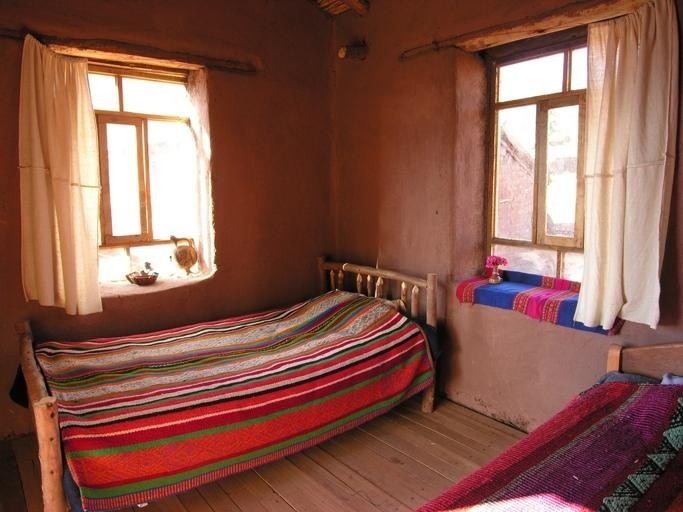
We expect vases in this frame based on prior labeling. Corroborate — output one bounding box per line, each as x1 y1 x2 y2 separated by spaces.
487 266 503 284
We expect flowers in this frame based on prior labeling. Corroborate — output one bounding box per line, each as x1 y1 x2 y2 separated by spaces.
483 254 507 269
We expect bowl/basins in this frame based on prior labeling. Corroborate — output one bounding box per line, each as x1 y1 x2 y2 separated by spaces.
126 271 158 286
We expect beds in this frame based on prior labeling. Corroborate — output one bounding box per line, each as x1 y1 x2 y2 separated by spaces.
413 339 682 512
12 249 441 512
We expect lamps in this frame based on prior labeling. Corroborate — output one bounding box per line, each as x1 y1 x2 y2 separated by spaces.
338 39 367 61
